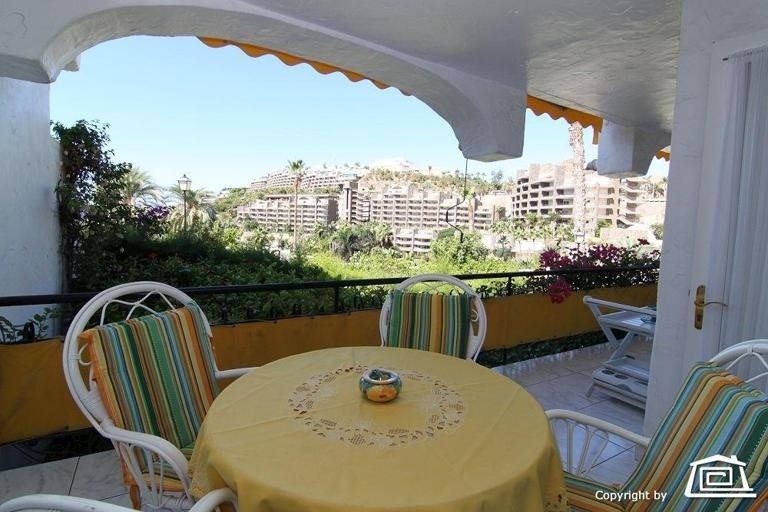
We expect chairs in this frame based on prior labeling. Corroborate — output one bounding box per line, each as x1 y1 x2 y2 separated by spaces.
0 468 250 512
57 278 266 512
374 270 486 363
531 336 765 510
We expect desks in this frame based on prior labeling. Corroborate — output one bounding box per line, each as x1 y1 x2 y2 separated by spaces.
182 342 572 512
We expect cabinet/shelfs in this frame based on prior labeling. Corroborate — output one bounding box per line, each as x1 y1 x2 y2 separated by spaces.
577 295 658 414
